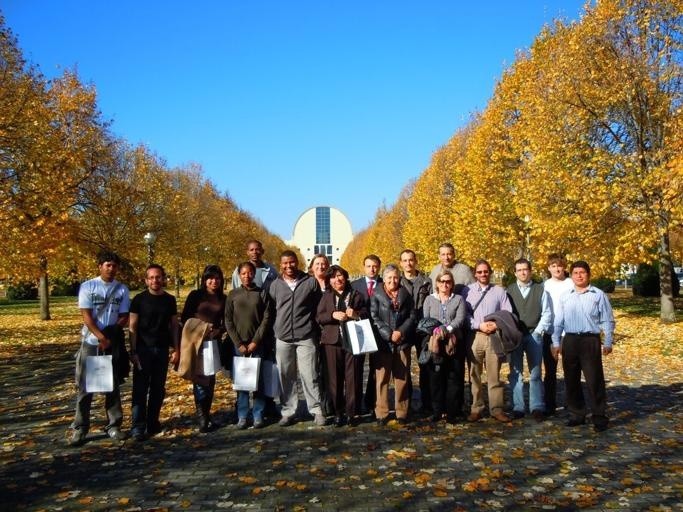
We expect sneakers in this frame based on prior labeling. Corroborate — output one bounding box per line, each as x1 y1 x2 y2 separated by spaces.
533 410 544 422
279 416 291 426
132 432 144 442
594 424 606 432
253 418 263 429
107 426 128 440
377 415 390 426
237 418 251 429
69 430 85 445
397 419 405 424
432 416 440 422
314 414 326 426
446 417 458 424
512 411 524 419
543 408 555 416
152 428 171 436
565 415 585 427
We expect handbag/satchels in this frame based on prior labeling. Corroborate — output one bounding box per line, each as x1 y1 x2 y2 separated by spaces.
203 339 222 376
86 347 114 393
74 345 84 386
232 352 262 392
346 316 379 355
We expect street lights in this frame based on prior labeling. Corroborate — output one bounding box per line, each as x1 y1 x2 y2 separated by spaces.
520 212 533 264
142 232 159 267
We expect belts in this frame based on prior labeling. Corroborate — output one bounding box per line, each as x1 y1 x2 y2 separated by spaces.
565 333 599 337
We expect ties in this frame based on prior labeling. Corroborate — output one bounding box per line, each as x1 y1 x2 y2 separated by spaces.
367 282 375 296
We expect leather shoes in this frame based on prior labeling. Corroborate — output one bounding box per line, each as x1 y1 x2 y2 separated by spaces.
467 414 480 422
334 415 342 427
347 415 354 427
491 413 510 423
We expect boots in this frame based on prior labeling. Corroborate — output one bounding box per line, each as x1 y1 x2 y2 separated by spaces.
208 419 215 432
196 404 208 433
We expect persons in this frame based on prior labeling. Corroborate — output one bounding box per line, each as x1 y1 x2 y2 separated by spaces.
316 265 365 427
129 264 180 436
308 254 328 293
72 252 129 443
350 254 384 411
539 254 582 416
422 270 465 425
270 249 328 426
461 260 513 424
552 261 615 432
231 241 278 290
399 249 432 356
371 263 415 427
503 258 554 423
174 265 227 432
225 261 273 429
428 243 476 296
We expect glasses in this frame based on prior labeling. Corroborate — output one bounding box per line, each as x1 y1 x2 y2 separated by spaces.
438 280 453 284
477 270 488 274
